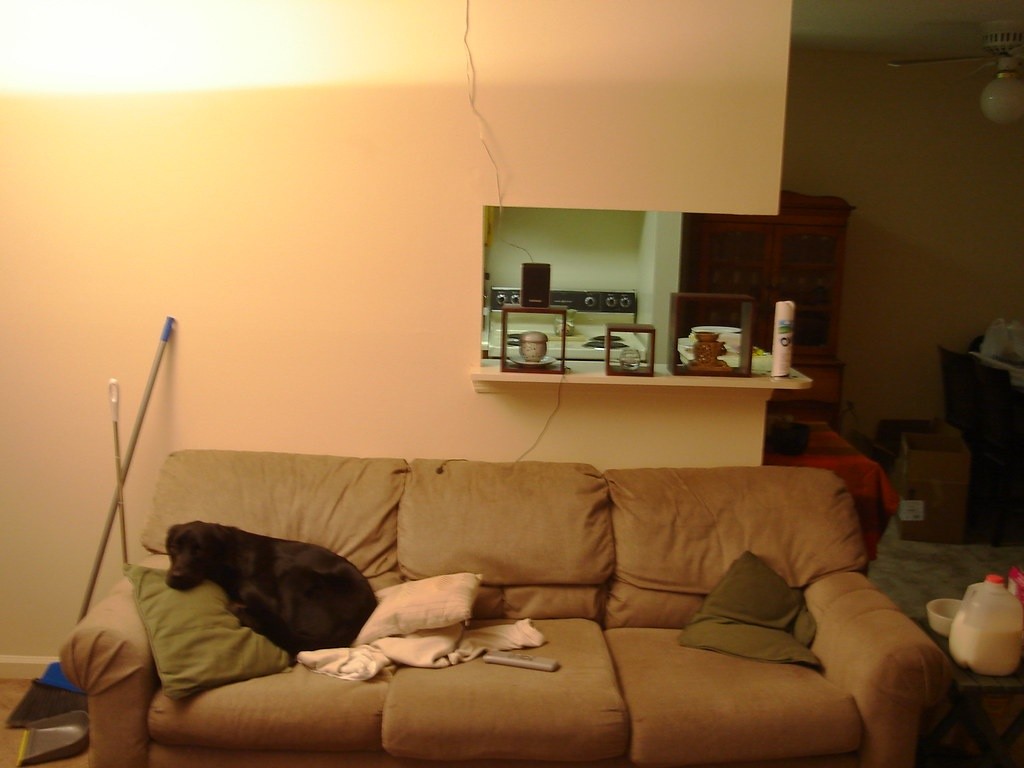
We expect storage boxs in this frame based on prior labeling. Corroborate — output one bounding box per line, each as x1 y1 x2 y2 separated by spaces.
857 418 971 544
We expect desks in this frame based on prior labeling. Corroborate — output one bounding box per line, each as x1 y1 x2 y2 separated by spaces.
763 422 900 578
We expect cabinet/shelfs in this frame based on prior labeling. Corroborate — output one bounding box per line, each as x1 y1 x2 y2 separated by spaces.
689 191 856 432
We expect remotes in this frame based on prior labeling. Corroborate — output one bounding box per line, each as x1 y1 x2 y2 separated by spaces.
484 649 559 670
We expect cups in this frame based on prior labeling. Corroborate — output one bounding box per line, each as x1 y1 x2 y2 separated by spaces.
619 349 641 371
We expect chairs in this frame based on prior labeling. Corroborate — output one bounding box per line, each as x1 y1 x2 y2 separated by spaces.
938 346 1024 547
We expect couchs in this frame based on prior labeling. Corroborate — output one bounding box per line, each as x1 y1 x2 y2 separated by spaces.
61 448 958 768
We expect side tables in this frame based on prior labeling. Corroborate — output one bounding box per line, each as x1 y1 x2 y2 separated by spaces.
912 615 1024 768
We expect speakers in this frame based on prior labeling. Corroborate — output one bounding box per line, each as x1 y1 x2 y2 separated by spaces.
520 264 550 308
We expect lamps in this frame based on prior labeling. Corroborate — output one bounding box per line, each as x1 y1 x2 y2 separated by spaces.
980 23 1024 124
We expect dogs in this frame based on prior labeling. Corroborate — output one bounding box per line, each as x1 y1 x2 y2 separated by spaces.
164 519 380 658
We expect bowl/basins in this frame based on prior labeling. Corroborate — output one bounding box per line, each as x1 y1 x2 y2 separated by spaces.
926 598 963 637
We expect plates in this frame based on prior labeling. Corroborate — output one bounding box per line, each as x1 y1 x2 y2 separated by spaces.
691 326 742 334
508 354 557 367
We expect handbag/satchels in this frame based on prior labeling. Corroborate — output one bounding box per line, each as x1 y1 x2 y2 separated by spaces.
982 318 1024 362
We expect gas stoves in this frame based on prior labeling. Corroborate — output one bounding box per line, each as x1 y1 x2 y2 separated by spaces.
486 329 647 362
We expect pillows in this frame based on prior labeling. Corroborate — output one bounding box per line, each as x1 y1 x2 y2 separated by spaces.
123 563 292 700
680 551 820 667
350 573 484 647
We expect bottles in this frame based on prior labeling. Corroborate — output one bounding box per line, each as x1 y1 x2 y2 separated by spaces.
947 573 1024 676
770 301 796 377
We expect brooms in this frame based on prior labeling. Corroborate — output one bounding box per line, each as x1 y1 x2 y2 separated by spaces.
5 315 176 731
